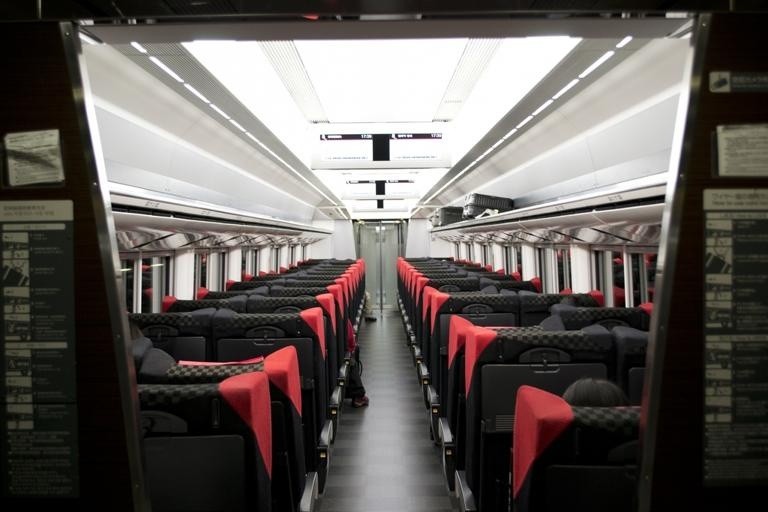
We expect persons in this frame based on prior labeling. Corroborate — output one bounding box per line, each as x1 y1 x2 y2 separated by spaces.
345 318 369 408
365 289 377 322
561 376 632 407
560 294 600 307
129 321 144 340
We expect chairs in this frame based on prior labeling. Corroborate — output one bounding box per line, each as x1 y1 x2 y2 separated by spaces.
127 255 367 512
395 255 654 512
